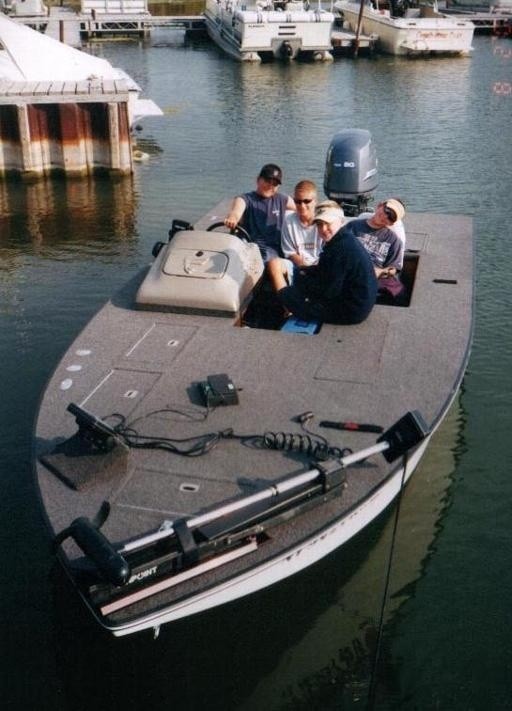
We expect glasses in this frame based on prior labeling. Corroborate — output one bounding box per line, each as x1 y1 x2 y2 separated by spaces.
294 198 312 204
384 206 396 222
264 177 279 186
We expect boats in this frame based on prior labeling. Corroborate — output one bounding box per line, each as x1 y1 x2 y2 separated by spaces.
31 127 477 639
203 0 477 64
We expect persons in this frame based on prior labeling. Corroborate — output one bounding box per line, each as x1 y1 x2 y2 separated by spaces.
266 181 322 291
223 164 298 268
270 199 379 326
336 198 403 305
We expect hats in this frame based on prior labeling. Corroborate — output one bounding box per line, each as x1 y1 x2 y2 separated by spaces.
383 199 405 226
260 164 282 185
310 200 344 225
